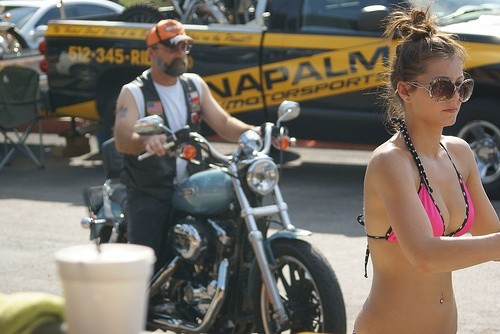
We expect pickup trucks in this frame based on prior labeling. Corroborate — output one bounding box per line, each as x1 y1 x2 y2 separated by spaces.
40 0 500 204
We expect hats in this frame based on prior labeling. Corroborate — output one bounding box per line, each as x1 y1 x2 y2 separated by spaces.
146 18 193 46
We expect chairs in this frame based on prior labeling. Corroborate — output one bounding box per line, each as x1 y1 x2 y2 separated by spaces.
0 64 43 168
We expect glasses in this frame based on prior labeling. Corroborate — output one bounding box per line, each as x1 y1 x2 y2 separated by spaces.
148 44 194 55
394 77 475 104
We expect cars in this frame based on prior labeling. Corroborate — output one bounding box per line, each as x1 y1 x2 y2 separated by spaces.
0 1 128 102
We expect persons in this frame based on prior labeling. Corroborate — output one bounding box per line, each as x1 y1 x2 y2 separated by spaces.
114 20 274 332
352 0 500 334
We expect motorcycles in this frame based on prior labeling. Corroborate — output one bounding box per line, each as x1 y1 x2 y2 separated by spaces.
78 100 349 334
